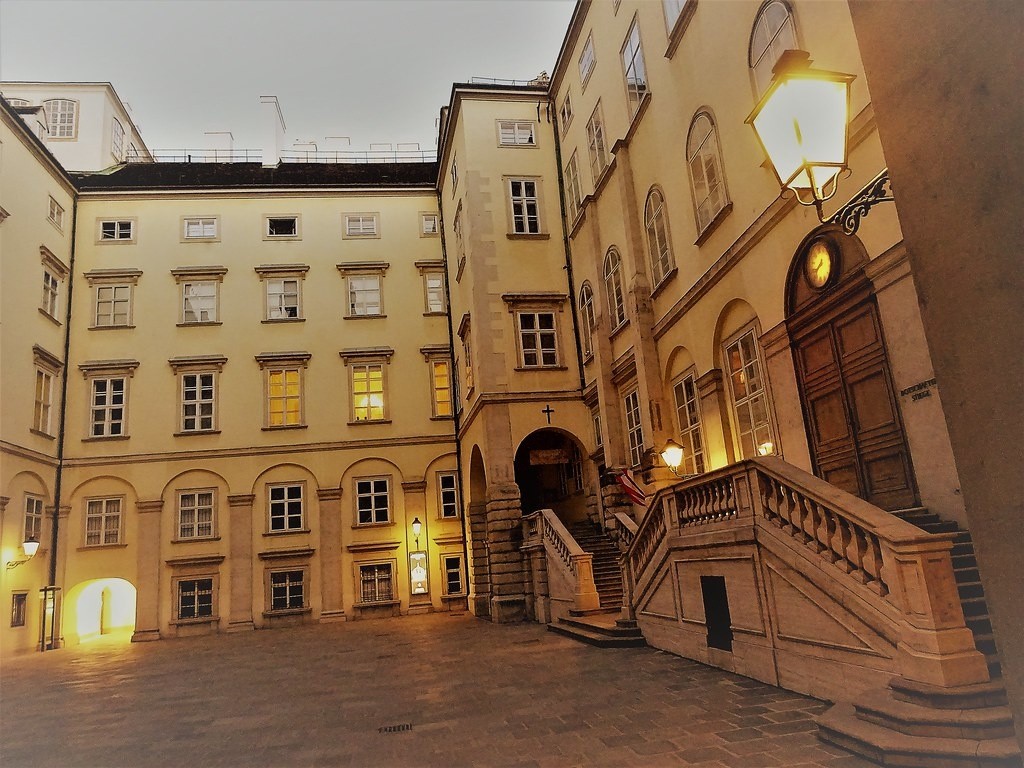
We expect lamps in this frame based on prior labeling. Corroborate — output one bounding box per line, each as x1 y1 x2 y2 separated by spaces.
742 47 859 206
659 438 685 474
412 517 422 537
22 535 40 560
